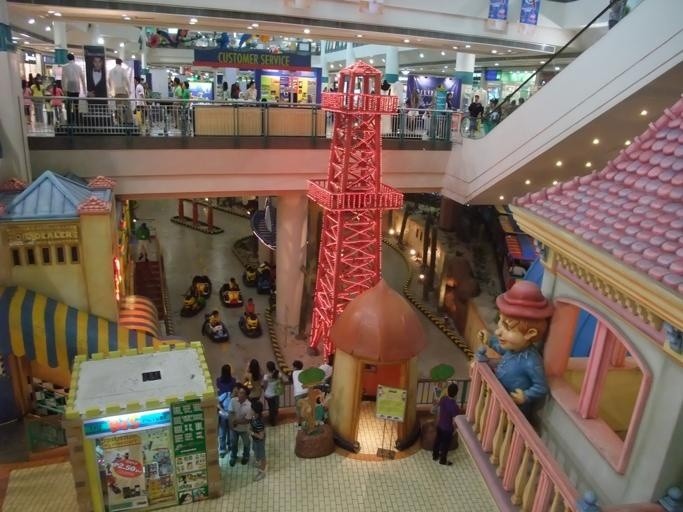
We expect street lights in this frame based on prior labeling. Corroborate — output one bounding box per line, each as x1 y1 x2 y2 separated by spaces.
274 382 284 396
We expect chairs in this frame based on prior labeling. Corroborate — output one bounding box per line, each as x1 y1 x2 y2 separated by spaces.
219 444 267 482
432 452 454 466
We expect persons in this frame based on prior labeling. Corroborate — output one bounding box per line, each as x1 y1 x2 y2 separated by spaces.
429 384 466 466
178 260 322 482
61 54 132 125
476 281 554 416
443 92 525 148
609 0 627 29
18 74 261 133
135 223 154 261
